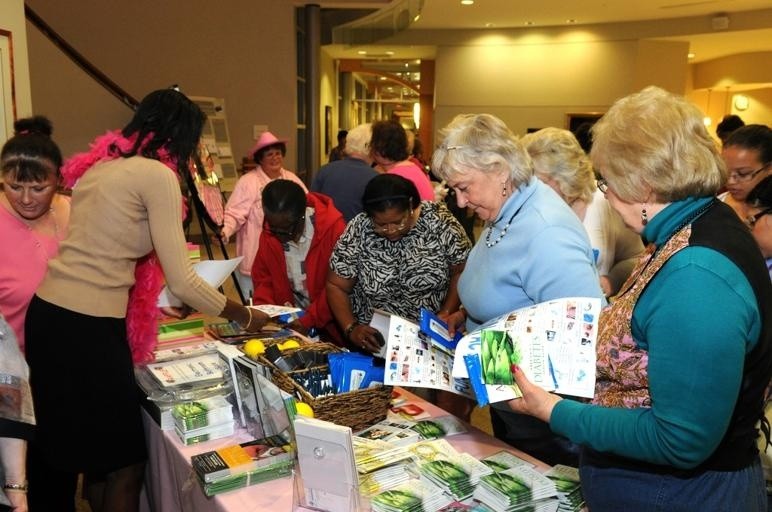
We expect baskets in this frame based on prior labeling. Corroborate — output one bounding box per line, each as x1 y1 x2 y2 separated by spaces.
259 342 393 430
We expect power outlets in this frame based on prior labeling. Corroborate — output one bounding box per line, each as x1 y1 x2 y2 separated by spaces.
253 125 268 140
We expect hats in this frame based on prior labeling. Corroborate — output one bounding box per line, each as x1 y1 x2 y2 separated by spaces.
248 132 288 160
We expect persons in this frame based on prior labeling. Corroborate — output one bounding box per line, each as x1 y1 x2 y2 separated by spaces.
0 315 36 512
251 179 346 348
432 113 609 468
0 116 72 359
326 175 472 402
521 128 645 297
744 174 772 284
511 88 772 512
213 131 309 300
370 121 435 202
25 89 269 512
312 123 381 225
329 131 347 162
717 124 772 221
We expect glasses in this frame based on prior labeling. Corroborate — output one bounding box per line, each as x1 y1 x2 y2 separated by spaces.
746 208 770 226
596 177 609 192
721 163 770 183
368 212 411 235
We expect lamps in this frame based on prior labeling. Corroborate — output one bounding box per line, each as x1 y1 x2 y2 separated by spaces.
719 86 730 123
703 89 712 125
413 103 420 129
735 96 749 110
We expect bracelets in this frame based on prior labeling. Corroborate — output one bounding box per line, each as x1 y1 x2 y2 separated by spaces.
440 307 454 314
346 320 359 342
242 307 252 330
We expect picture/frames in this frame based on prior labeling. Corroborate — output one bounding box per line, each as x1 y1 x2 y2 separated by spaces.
0 28 18 153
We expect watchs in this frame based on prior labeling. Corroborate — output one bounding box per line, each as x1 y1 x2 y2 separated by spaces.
4 483 28 491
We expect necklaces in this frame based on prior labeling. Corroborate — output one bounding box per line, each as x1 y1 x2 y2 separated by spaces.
618 200 715 299
486 204 523 248
23 207 60 262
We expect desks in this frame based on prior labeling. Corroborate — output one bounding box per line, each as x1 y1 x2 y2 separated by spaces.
130 311 589 512
